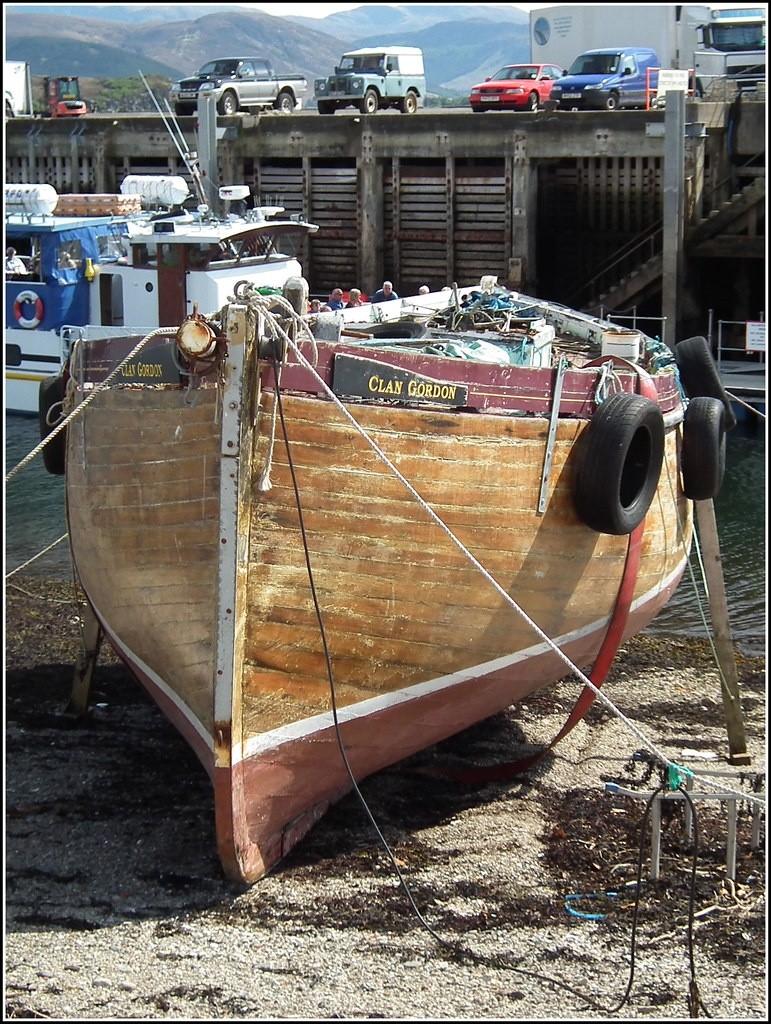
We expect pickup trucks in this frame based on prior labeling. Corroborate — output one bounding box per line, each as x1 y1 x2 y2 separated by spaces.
169 57 309 116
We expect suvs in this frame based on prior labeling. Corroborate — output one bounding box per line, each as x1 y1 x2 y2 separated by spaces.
313 46 426 114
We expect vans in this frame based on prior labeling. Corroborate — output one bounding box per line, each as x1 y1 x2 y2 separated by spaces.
549 46 661 110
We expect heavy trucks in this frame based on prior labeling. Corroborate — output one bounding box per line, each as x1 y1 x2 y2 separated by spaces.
529 2 768 99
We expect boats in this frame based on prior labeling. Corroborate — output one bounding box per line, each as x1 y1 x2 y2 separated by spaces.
6 174 318 414
64 277 695 888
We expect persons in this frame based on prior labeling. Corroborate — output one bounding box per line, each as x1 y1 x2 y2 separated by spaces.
307 289 362 314
57 252 75 269
371 281 398 305
6 248 26 280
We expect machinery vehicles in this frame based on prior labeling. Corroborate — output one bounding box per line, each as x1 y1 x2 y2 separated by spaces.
32 76 87 118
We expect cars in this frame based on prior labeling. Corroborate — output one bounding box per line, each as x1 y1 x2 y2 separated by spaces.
467 64 568 112
